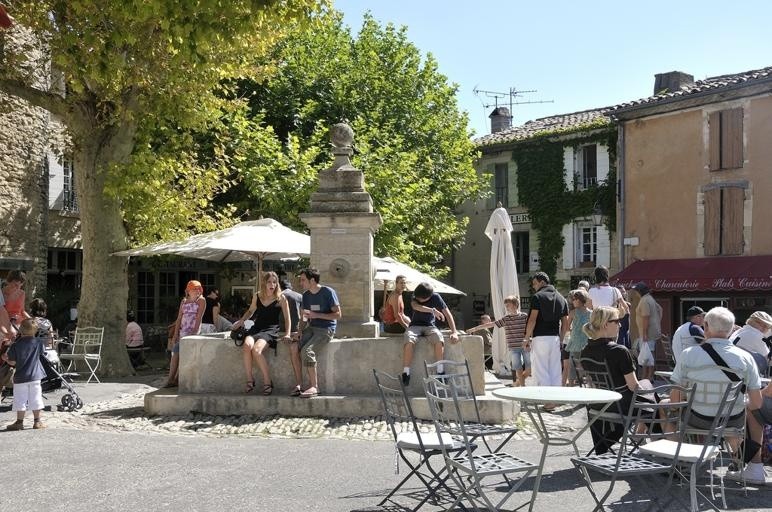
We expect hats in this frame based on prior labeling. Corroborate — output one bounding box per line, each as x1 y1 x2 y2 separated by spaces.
186 280 203 292
688 306 707 316
750 310 772 325
631 281 648 292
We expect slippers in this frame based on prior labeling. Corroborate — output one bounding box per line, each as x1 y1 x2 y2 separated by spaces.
538 407 555 411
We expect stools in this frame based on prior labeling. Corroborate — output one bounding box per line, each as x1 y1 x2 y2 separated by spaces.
127 346 151 368
485 354 492 371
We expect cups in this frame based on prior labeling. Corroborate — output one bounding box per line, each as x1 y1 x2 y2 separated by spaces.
302 309 311 322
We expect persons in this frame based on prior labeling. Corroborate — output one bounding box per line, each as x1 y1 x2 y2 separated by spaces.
0 269 78 431
383 274 411 333
521 263 629 387
126 310 146 367
288 266 342 398
162 279 207 388
218 305 255 331
631 283 663 384
279 279 303 332
465 294 532 388
580 305 662 450
400 281 459 387
200 286 221 334
473 314 493 370
657 306 771 486
232 270 293 396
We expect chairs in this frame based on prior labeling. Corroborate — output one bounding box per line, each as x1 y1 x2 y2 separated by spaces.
58 326 105 383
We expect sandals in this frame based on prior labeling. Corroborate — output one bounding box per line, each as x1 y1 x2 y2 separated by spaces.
244 381 255 393
263 379 273 396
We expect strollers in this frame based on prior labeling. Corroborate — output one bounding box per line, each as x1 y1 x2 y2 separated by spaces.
39 355 84 412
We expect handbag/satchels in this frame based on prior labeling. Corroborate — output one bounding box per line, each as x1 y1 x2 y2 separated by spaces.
230 324 248 346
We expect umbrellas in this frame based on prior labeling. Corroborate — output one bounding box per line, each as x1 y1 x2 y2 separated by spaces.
484 200 521 375
109 216 392 296
374 256 467 297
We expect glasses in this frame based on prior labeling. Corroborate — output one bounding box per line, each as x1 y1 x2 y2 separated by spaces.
214 291 220 297
607 318 620 324
14 278 25 283
573 299 577 301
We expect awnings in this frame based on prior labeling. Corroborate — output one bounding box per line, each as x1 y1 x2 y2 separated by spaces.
609 254 772 290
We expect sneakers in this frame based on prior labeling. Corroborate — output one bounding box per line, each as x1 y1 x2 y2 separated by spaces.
726 461 765 481
436 370 448 384
402 372 410 386
288 386 304 396
6 422 23 429
300 386 321 398
32 422 46 429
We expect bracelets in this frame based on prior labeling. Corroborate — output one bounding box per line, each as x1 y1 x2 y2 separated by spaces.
432 307 436 316
